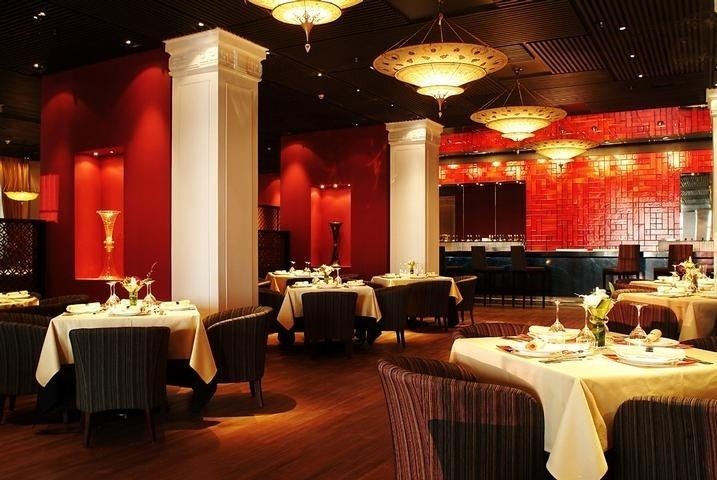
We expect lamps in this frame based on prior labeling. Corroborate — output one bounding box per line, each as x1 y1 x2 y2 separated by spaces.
246 0 365 53
470 65 567 155
4 157 39 205
525 117 600 172
373 0 507 117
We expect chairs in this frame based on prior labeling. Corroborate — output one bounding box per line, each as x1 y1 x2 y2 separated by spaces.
613 395 717 480
612 288 651 302
376 356 544 480
602 245 640 289
8 294 89 317
679 338 717 352
511 246 535 309
451 321 530 351
606 300 679 341
204 305 273 409
69 327 170 448
654 244 693 280
0 313 54 426
408 281 452 332
472 246 502 307
258 283 285 310
614 279 658 292
347 279 384 291
375 285 408 349
301 292 358 359
438 276 478 329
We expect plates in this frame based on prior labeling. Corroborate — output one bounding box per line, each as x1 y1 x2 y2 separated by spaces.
615 345 684 368
515 341 589 358
625 336 680 347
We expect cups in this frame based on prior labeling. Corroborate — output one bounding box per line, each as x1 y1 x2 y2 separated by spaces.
142 281 153 308
307 262 311 271
112 282 121 304
335 268 342 287
547 298 567 343
289 261 296 273
575 304 596 350
672 265 679 275
106 282 117 307
147 280 157 302
670 271 679 287
304 262 308 271
630 303 648 339
